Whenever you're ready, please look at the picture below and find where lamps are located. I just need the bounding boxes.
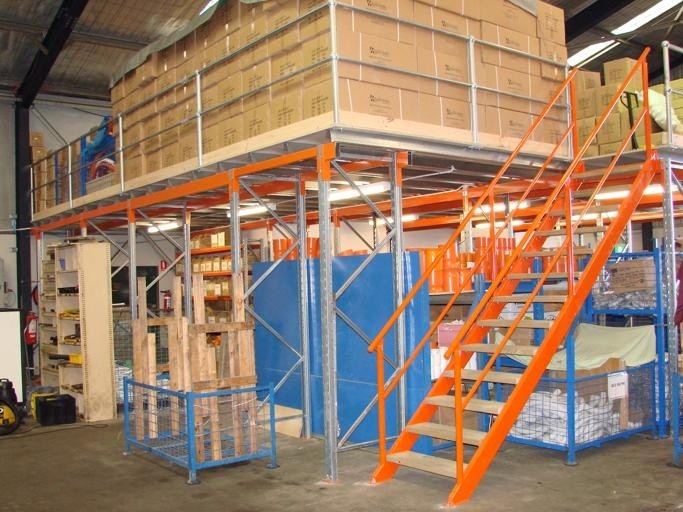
[470,218,527,231]
[326,180,392,202]
[592,182,680,202]
[570,208,621,223]
[473,198,531,214]
[148,218,184,233]
[225,200,277,219]
[368,213,421,227]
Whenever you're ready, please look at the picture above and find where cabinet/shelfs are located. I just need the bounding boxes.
[494,248,683,465]
[39,242,118,422]
[176,243,261,301]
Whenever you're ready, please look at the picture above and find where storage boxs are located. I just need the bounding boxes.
[550,354,683,423]
[30,0,683,211]
[31,390,77,426]
[174,227,257,324]
[609,256,656,295]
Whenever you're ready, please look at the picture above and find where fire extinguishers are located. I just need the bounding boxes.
[160,290,171,315]
[24,311,38,345]
